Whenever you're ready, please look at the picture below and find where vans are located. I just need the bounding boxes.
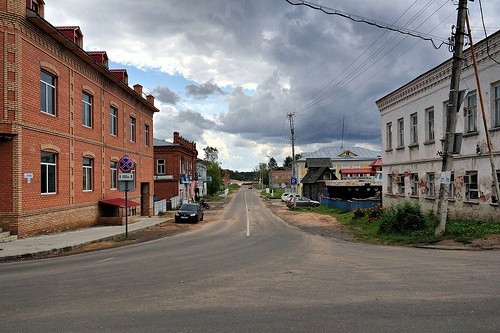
[281,193,300,201]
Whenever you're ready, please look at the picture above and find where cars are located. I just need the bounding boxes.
[174,204,204,224]
[248,186,252,189]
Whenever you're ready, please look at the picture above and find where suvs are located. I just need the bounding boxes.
[287,197,321,210]
